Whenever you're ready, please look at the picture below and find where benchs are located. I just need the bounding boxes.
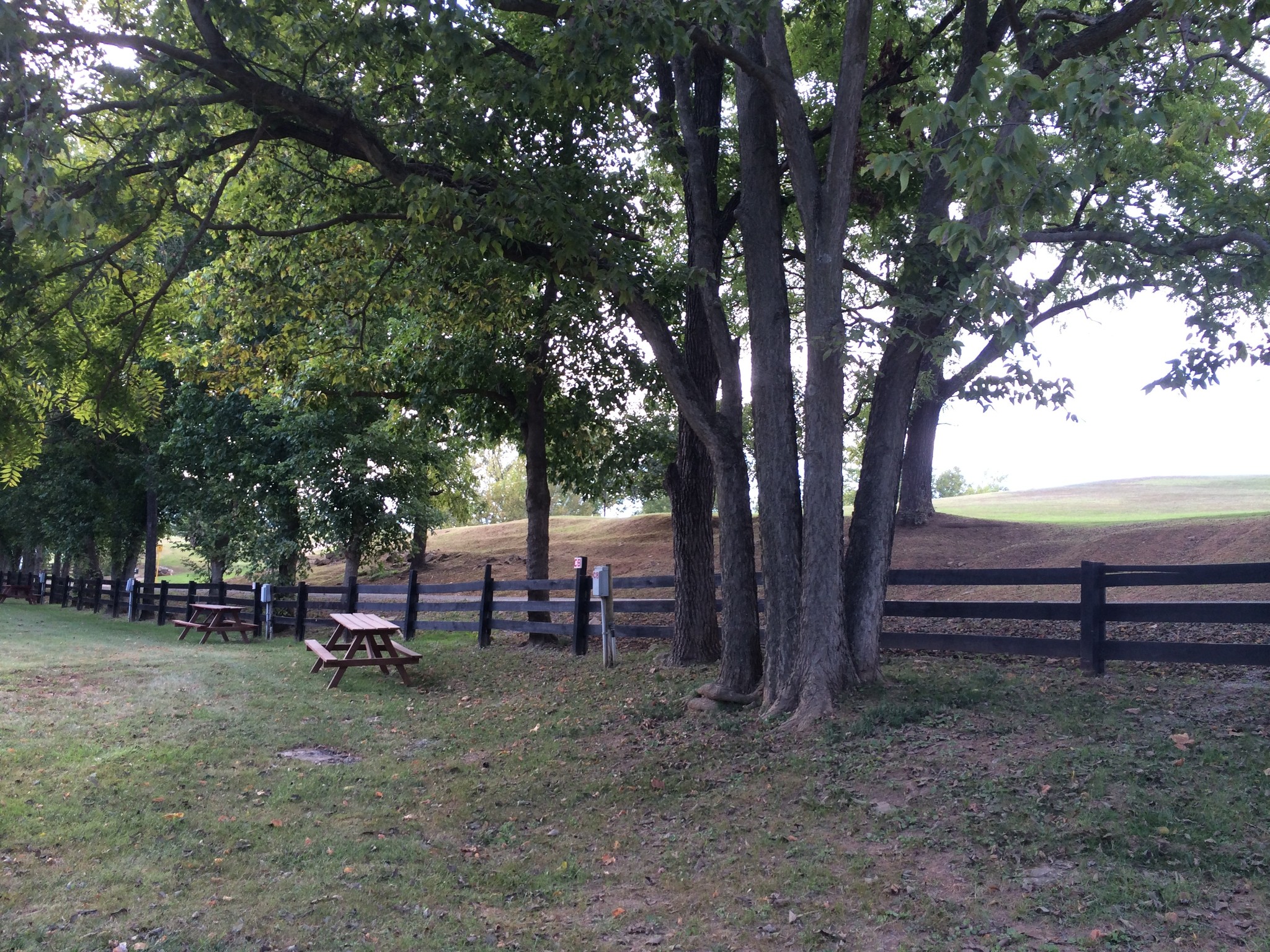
[0,592,8,598]
[171,619,208,632]
[388,640,423,661]
[302,639,341,667]
[31,592,42,598]
[222,619,260,630]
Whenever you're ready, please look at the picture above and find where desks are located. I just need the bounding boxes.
[0,584,38,605]
[310,610,412,691]
[178,603,250,646]
